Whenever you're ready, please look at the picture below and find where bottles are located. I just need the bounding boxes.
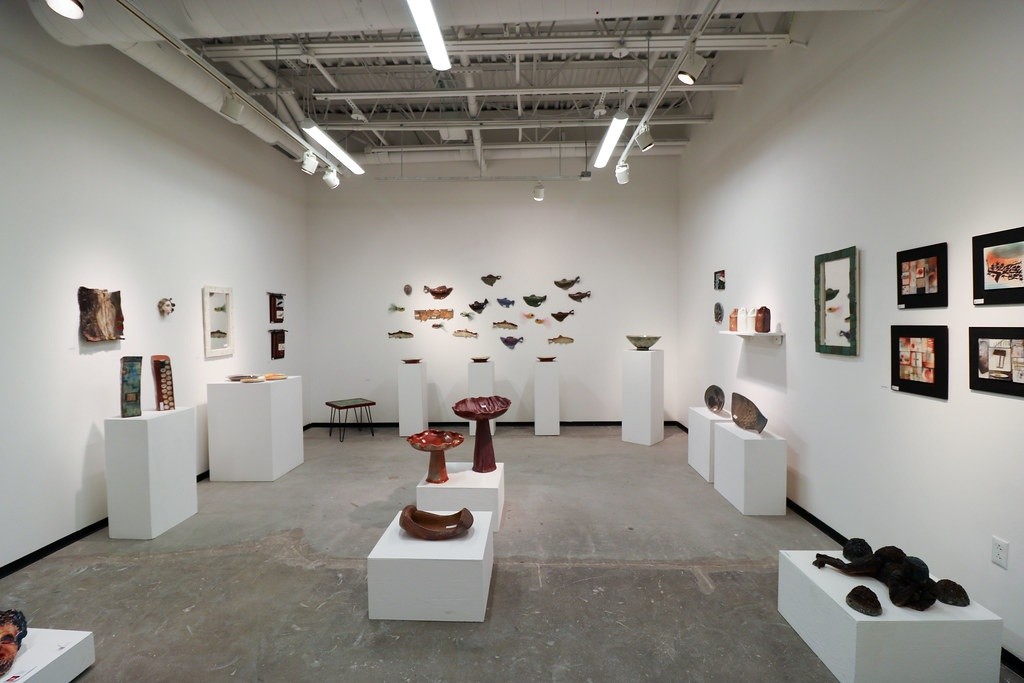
[729,306,770,333]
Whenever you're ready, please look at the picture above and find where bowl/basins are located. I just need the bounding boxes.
[626,335,662,351]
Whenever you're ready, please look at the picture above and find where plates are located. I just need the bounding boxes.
[704,385,725,413]
[226,373,288,382]
[537,356,556,362]
[402,358,421,364]
[471,357,489,363]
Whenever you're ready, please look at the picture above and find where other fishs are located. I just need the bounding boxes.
[547,335,574,345]
[569,291,592,302]
[500,336,524,348]
[524,312,545,324]
[481,274,501,287]
[496,297,515,308]
[388,304,405,313]
[493,320,519,330]
[553,276,579,290]
[453,330,478,338]
[522,294,547,307]
[468,299,489,314]
[387,330,413,339]
[424,285,452,296]
[432,324,443,330]
[551,310,574,323]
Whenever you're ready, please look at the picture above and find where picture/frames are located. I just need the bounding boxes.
[891,225,1023,400]
[814,246,859,356]
[203,286,236,358]
[713,270,725,290]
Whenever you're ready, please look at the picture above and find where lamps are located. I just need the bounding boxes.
[322,169,340,191]
[676,41,709,84]
[615,162,630,186]
[534,180,545,201]
[220,93,244,122]
[46,0,84,20]
[406,0,452,72]
[586,52,630,172]
[302,150,319,176]
[298,64,366,176]
[634,125,656,152]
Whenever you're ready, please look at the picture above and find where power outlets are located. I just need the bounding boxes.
[991,534,1010,570]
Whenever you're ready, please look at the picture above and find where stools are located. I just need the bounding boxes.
[325,398,376,442]
